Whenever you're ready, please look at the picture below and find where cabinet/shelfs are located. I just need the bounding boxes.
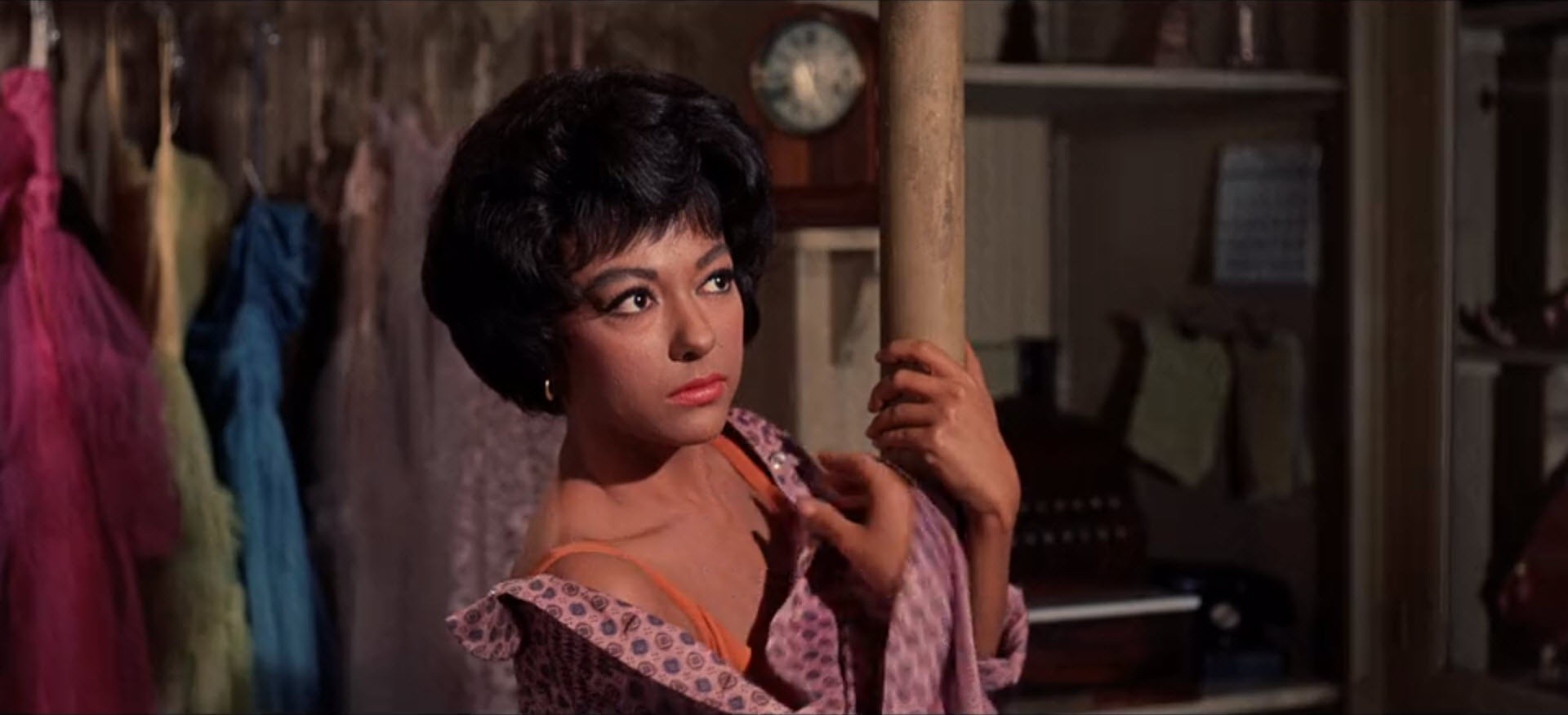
[969,52,1352,715]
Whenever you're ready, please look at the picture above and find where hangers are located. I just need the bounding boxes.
[19,0,624,230]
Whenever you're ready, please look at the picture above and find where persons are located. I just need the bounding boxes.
[421,59,1039,715]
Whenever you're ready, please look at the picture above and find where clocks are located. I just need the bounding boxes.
[731,16,889,198]
